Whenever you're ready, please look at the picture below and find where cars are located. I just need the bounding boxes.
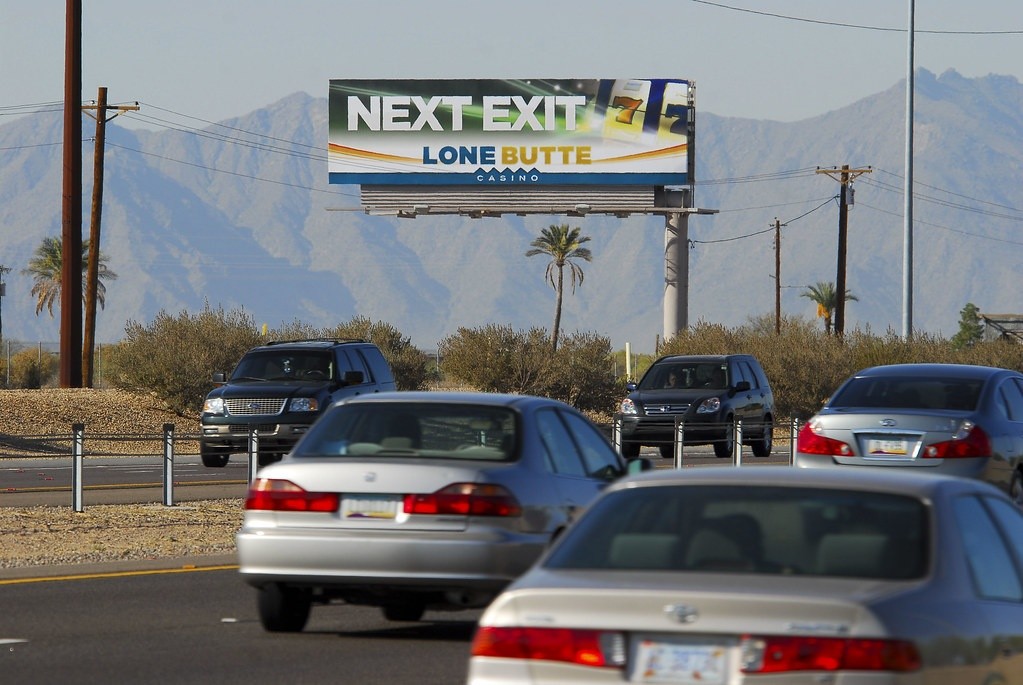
[470,469,1022,685]
[234,391,652,633]
[794,364,1023,489]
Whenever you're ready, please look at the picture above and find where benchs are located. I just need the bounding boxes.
[323,438,512,463]
[607,529,922,577]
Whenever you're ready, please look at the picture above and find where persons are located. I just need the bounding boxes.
[265,360,285,380]
[704,367,725,388]
[666,370,684,388]
[895,387,922,409]
[295,356,328,378]
[942,382,972,410]
[391,414,423,448]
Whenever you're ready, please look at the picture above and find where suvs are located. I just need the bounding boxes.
[614,354,775,461]
[199,338,400,469]
